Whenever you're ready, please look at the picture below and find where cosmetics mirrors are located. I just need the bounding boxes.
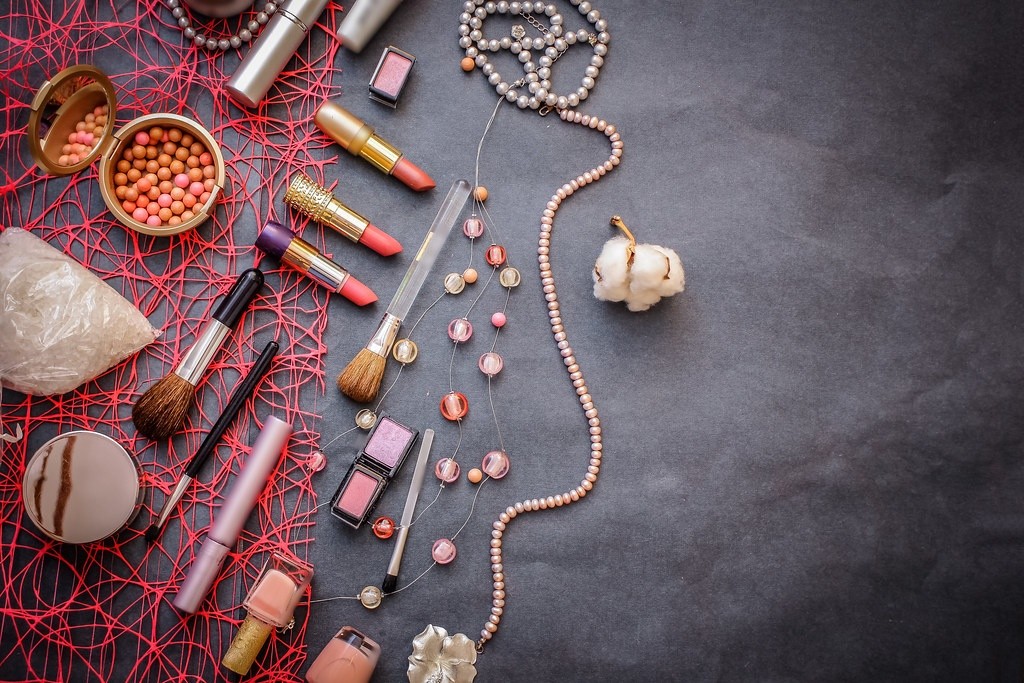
[28,65,116,177]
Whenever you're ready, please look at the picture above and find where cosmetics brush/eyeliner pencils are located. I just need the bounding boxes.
[336,179,472,403]
[381,428,435,594]
[132,267,280,545]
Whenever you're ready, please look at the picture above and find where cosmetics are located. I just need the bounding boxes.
[23,431,146,545]
[226,0,418,110]
[172,415,419,683]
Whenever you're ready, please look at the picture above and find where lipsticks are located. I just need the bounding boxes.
[254,102,437,307]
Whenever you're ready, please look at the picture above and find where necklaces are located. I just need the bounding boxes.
[306,0,624,683]
[166,0,284,49]
